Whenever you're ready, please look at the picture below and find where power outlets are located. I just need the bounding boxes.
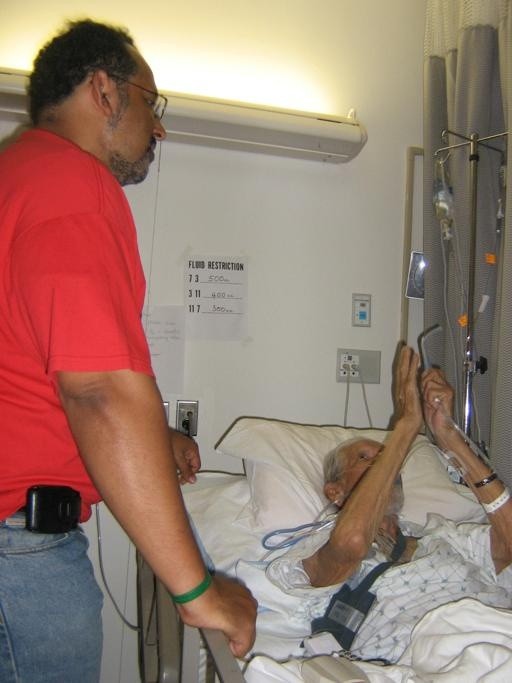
[336,348,382,386]
[176,400,200,438]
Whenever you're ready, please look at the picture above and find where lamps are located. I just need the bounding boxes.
[0,61,367,166]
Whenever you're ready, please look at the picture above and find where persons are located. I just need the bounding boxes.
[0,20,258,683]
[266,345,512,683]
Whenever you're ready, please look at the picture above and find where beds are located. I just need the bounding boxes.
[133,412,511,682]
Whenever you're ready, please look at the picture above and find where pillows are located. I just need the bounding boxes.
[214,416,491,537]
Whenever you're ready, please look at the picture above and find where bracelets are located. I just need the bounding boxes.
[172,570,213,604]
[475,472,497,487]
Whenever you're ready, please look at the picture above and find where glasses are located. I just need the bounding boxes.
[108,71,167,120]
